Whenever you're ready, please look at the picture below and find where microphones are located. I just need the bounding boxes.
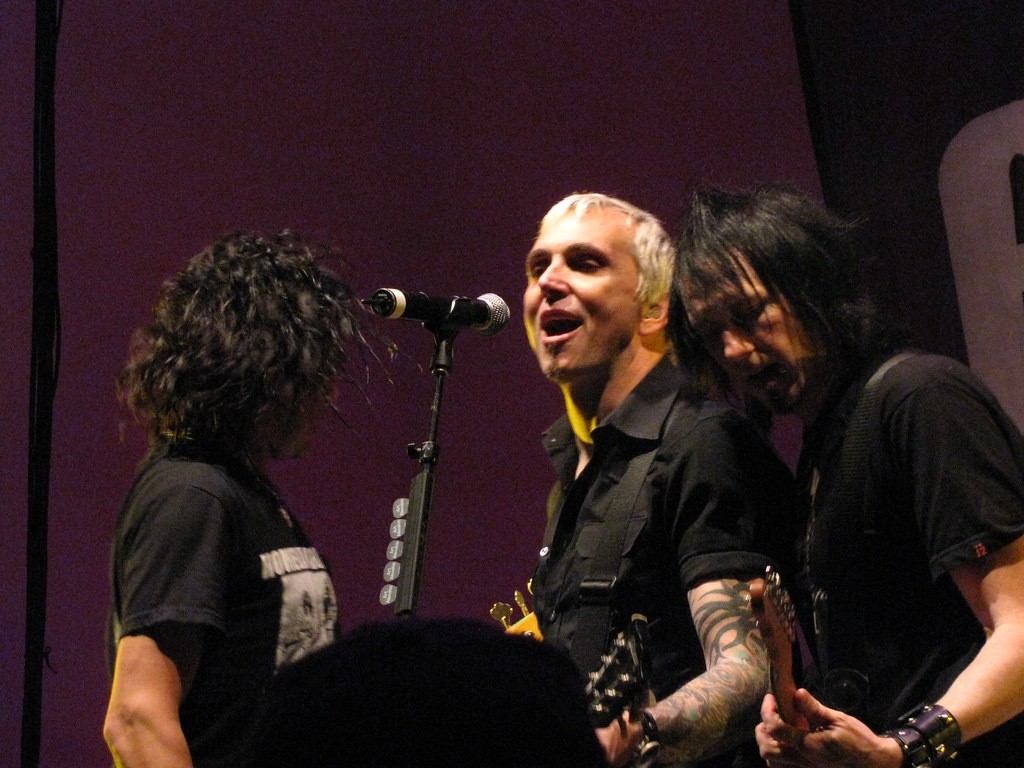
[361,289,511,336]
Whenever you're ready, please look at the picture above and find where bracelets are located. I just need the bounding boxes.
[883,703,961,768]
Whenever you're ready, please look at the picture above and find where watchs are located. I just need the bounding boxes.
[635,710,661,768]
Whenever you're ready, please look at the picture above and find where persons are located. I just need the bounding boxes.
[663,180,1024,768]
[521,192,794,768]
[101,228,370,768]
[250,616,605,768]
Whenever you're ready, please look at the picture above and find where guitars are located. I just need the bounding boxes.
[748,564,823,732]
[579,613,649,726]
